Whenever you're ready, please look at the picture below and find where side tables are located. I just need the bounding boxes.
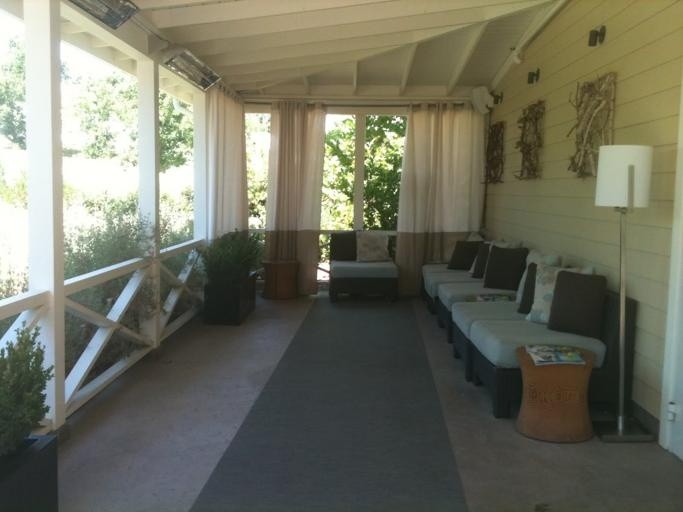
[514,345,596,443]
[260,260,300,299]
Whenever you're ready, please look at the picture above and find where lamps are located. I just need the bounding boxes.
[161,43,222,92]
[528,69,540,84]
[592,145,655,443]
[589,26,606,46]
[494,92,503,104]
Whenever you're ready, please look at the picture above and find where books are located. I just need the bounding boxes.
[525,342,587,369]
[475,291,510,303]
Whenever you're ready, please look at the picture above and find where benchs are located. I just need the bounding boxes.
[330,231,397,302]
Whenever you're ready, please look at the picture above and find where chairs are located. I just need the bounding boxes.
[470,272,637,418]
[451,252,595,382]
[424,232,531,347]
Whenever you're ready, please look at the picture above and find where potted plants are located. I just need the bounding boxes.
[203,229,267,324]
[0,320,57,512]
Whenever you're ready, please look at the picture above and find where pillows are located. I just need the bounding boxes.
[356,232,390,261]
[516,262,538,315]
[526,262,593,325]
[525,249,562,270]
[545,271,610,341]
